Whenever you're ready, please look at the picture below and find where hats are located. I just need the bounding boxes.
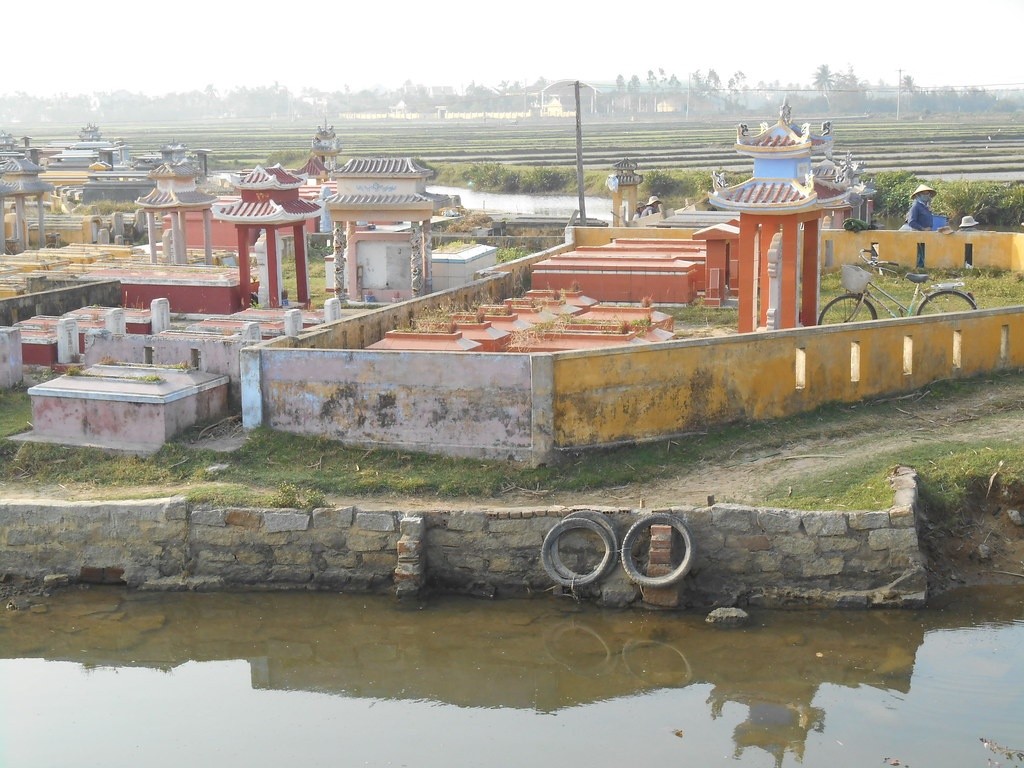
[636,202,645,212]
[645,196,663,207]
[959,215,979,227]
[905,212,909,221]
[911,184,936,199]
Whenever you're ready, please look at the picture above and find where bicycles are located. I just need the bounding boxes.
[816,249,978,327]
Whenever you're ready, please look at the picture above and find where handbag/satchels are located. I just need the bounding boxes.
[842,218,871,233]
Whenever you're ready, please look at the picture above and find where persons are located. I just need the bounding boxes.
[706,200,718,211]
[632,194,663,221]
[899,183,937,230]
[958,215,979,231]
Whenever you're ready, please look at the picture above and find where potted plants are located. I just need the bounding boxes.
[392,291,402,303]
[281,289,288,307]
[364,290,374,302]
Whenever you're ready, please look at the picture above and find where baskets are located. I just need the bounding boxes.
[841,264,872,292]
